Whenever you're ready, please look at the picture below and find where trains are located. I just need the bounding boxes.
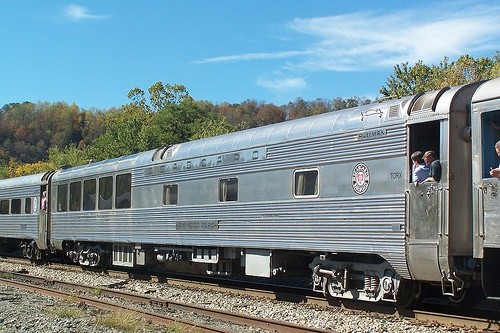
[0,76,500,308]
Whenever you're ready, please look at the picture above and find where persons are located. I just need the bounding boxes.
[489,140,500,178]
[41,191,46,210]
[410,152,431,187]
[423,151,442,184]
